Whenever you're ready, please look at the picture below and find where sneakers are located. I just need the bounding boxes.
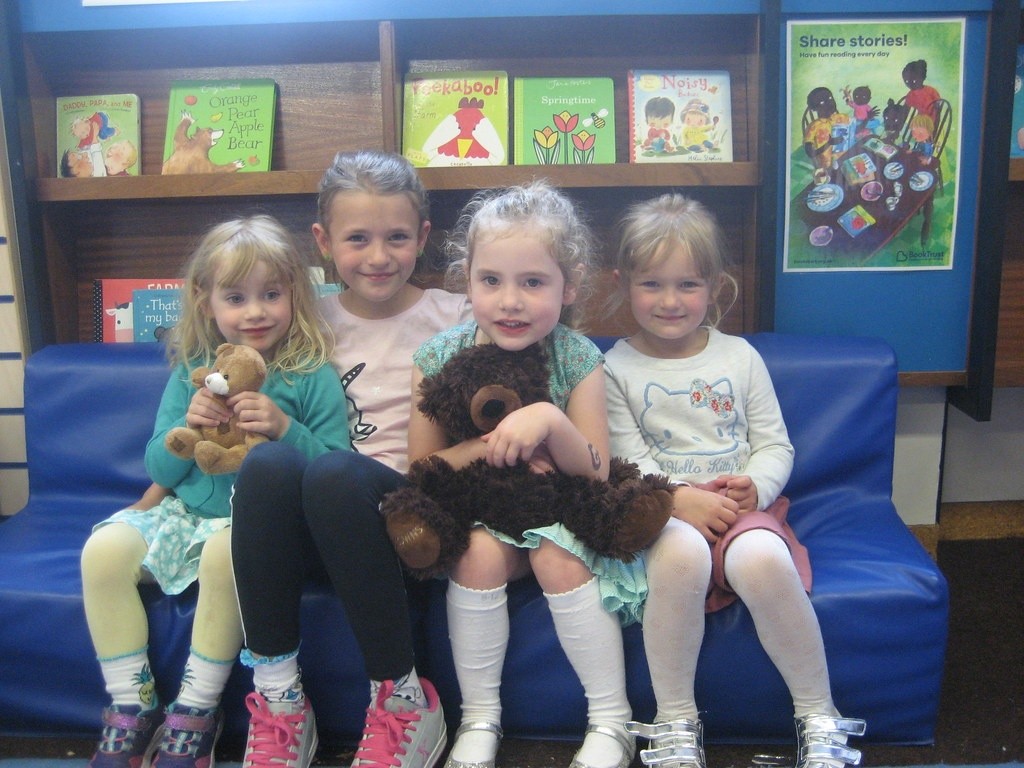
[350,675,448,768]
[624,719,707,768]
[242,690,318,768]
[87,702,167,768]
[794,704,867,768]
[151,702,226,768]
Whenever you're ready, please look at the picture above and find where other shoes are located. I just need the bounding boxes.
[442,721,504,768]
[569,724,637,768]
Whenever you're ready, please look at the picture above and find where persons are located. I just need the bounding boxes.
[598,193,868,768]
[78,214,348,768]
[231,147,475,768]
[406,177,636,768]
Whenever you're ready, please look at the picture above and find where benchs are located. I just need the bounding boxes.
[0,330,954,752]
